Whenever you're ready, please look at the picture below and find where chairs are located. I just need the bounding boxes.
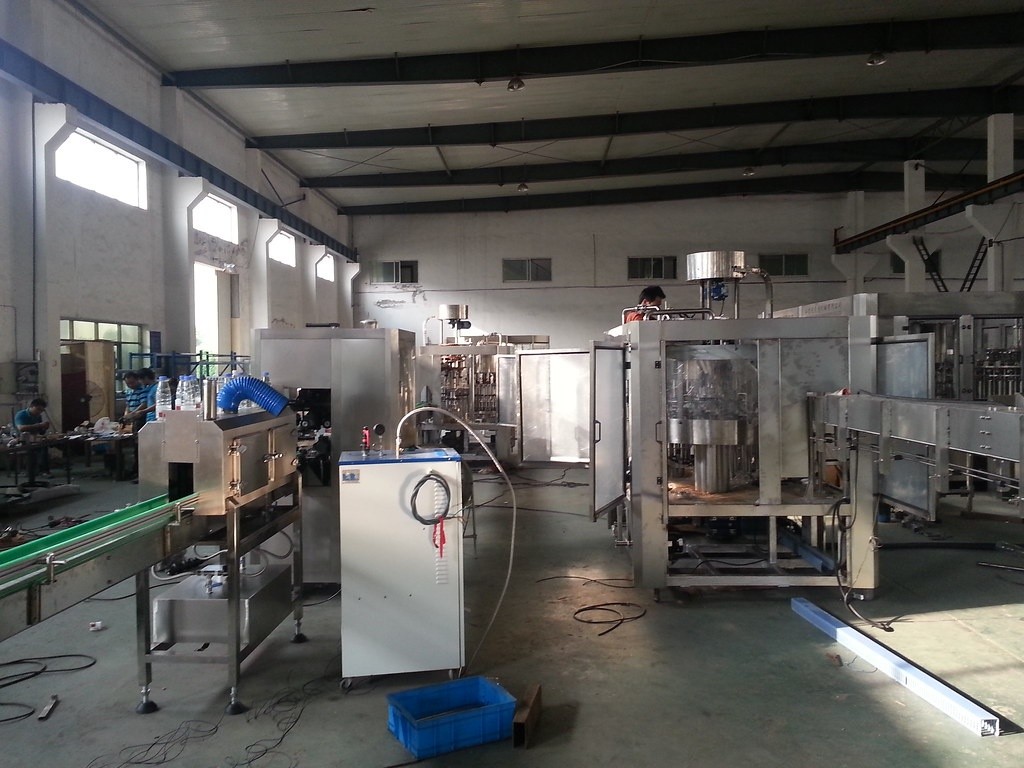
[5,406,51,484]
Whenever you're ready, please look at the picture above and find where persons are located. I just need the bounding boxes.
[14,398,54,479]
[625,285,666,323]
[118,368,159,484]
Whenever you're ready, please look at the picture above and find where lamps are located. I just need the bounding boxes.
[518,184,529,192]
[507,79,525,92]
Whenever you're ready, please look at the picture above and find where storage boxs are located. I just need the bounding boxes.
[386,675,517,760]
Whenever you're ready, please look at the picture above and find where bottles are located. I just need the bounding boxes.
[176,375,201,410]
[155,376,172,420]
[224,371,271,409]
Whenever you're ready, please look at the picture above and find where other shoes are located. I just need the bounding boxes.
[130,472,138,479]
[42,472,54,478]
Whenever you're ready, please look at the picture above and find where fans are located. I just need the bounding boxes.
[64,380,104,421]
